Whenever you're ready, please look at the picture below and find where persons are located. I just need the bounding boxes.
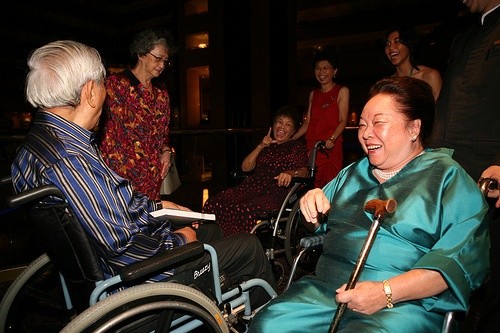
[199,108,310,235]
[99,29,174,200]
[0,0,500,333]
[291,56,349,188]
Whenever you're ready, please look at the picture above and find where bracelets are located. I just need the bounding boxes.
[292,168,298,177]
[329,136,335,142]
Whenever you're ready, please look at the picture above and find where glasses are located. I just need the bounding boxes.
[314,66,333,72]
[145,48,170,66]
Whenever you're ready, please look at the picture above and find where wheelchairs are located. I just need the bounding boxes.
[227,140,333,274]
[285,178,499,333]
[0,175,281,333]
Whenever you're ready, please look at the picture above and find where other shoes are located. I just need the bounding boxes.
[273,261,284,286]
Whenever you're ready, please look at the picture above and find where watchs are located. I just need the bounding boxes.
[162,150,173,160]
[382,278,394,308]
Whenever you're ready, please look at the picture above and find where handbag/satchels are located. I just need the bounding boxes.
[160,150,182,195]
